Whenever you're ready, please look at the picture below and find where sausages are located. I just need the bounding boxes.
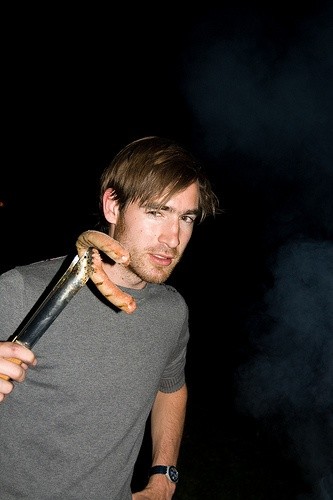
[75,229,139,315]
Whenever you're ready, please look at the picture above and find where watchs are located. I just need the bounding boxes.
[149,464,182,484]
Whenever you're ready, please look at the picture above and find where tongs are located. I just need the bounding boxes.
[0,247,95,380]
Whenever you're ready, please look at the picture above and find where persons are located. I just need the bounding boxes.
[1,134,225,500]
